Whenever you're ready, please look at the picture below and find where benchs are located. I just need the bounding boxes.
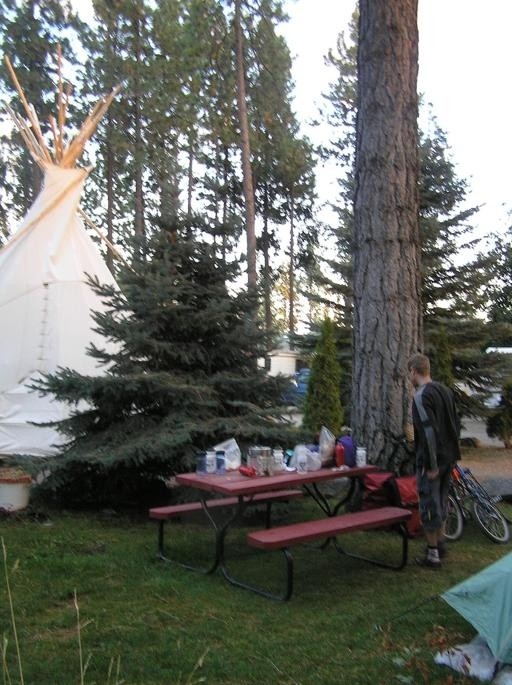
[242,507,418,603]
[148,489,311,575]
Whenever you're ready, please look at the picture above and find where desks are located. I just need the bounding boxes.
[173,460,380,576]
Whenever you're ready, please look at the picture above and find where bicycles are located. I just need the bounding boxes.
[399,437,511,545]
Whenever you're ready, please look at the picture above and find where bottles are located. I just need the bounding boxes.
[196,425,367,475]
[452,468,460,480]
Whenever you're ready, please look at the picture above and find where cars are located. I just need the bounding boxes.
[277,368,311,407]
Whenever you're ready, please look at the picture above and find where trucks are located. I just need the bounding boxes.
[454,346,512,449]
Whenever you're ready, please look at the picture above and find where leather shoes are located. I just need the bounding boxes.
[419,541,447,558]
[413,554,442,570]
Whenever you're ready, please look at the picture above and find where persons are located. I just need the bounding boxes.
[406,355,462,568]
